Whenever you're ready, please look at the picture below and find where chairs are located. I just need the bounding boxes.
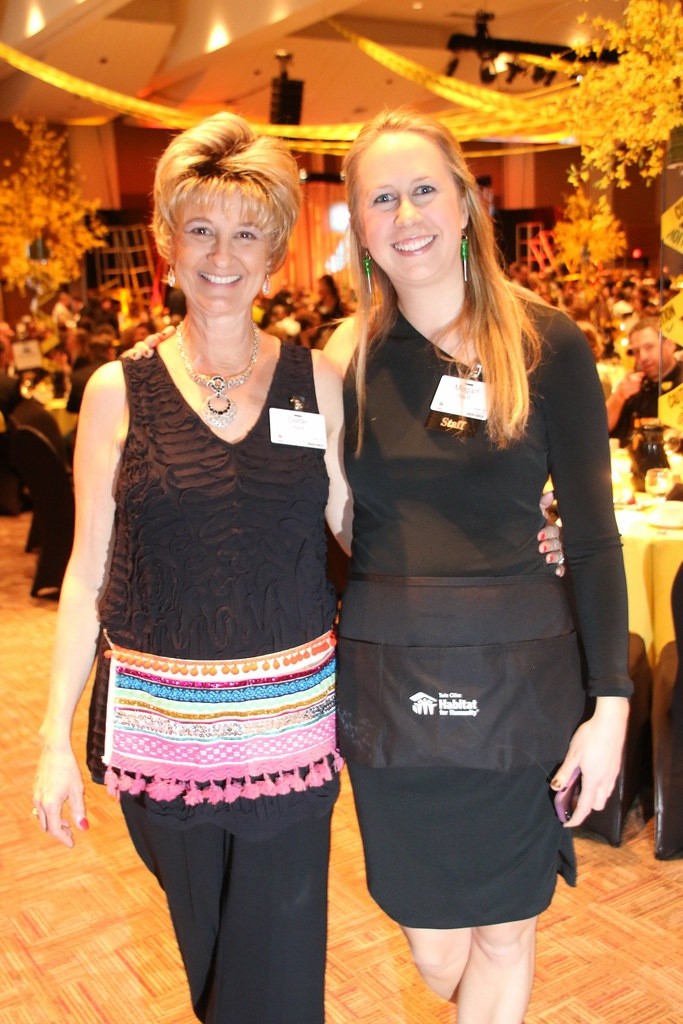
[4,423,74,598]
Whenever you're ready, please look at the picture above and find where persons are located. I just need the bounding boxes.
[0,271,682,599]
[321,113,636,1024]
[31,111,573,1022]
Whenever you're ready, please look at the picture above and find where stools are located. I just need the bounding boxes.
[646,641,683,859]
[576,632,653,847]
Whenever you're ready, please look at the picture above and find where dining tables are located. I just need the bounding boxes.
[607,490,682,669]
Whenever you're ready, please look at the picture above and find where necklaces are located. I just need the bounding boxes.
[173,320,259,424]
